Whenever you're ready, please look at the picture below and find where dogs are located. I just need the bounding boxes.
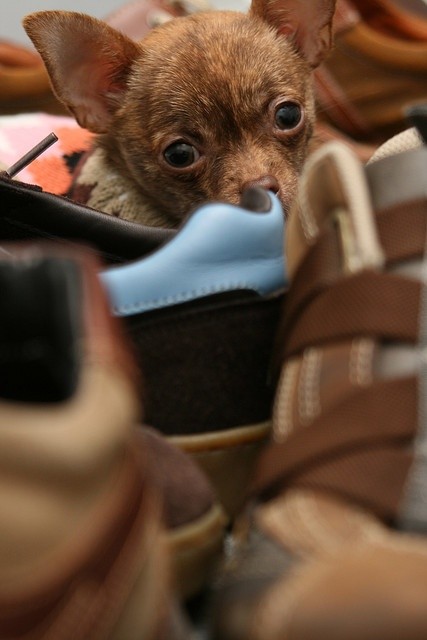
[23,2,339,227]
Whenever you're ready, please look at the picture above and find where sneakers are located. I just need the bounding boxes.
[0,131,287,526]
[0,248,229,639]
[212,126,426,638]
